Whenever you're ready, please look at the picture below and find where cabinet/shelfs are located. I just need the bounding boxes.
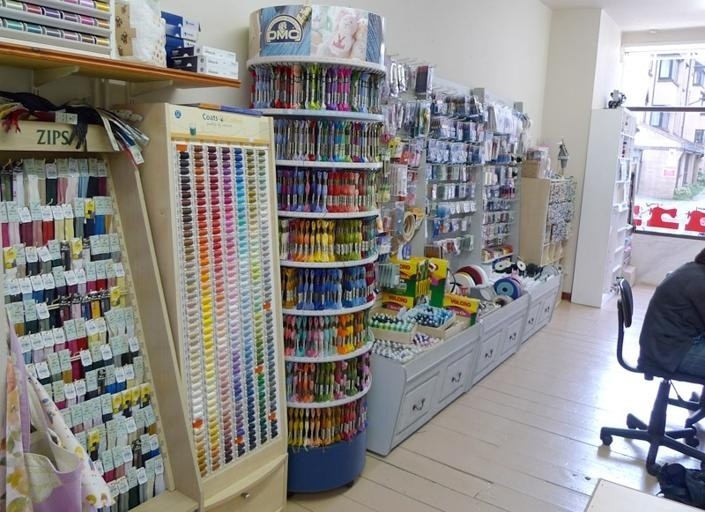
[359,273,563,459]
[519,106,639,309]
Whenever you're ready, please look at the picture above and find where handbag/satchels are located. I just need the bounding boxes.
[656,464,705,509]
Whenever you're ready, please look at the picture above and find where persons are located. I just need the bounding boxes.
[636,248,705,377]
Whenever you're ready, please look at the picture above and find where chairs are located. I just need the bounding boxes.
[599,278,705,478]
